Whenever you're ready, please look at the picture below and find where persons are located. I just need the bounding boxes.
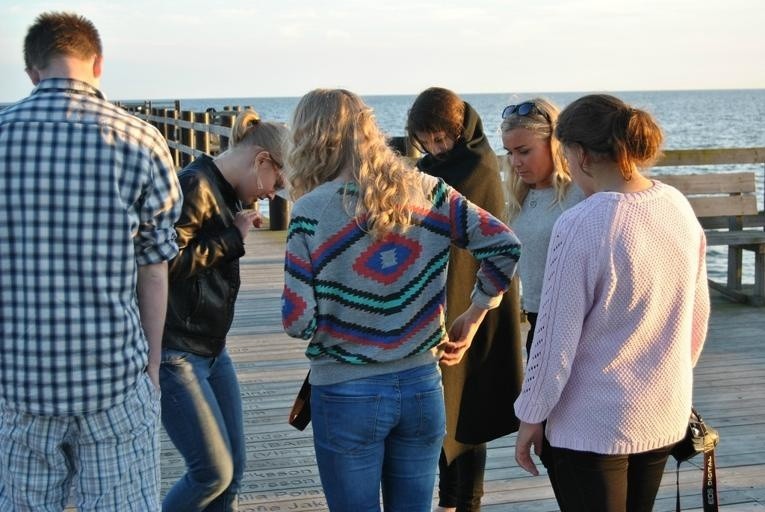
[160,107,293,509]
[280,90,524,512]
[408,89,525,512]
[513,94,710,512]
[0,10,180,510]
[499,98,588,365]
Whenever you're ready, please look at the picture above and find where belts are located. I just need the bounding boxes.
[289,368,311,430]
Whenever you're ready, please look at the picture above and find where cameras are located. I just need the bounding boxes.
[674,418,719,464]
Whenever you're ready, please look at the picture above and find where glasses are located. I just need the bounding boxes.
[502,103,543,119]
